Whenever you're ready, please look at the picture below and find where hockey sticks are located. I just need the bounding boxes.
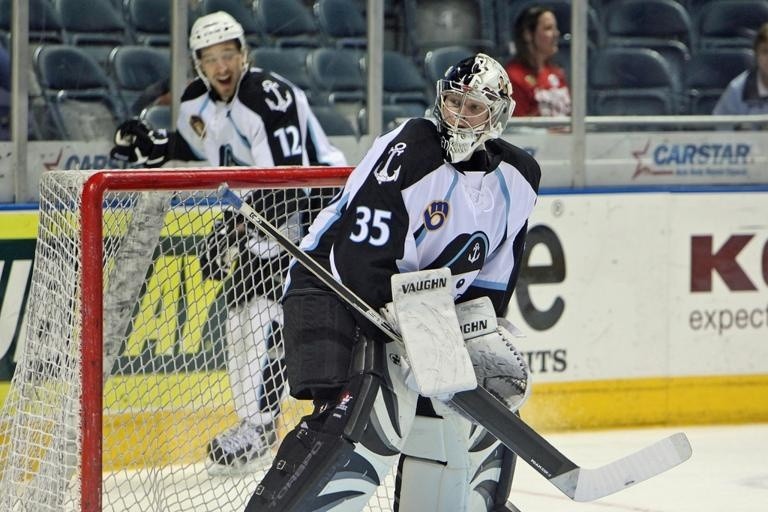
[219,183,693,502]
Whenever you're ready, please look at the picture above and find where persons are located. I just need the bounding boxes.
[113,11,348,465]
[711,24,768,130]
[243,53,542,512]
[504,5,571,119]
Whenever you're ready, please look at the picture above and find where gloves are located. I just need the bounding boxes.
[201,210,255,282]
[110,118,169,168]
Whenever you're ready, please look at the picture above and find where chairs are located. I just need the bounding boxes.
[585,0,767,132]
[383,1,573,137]
[1,0,369,137]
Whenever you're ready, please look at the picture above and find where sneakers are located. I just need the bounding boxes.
[207,417,277,467]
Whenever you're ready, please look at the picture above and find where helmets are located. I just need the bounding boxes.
[188,11,247,93]
[432,53,516,164]
